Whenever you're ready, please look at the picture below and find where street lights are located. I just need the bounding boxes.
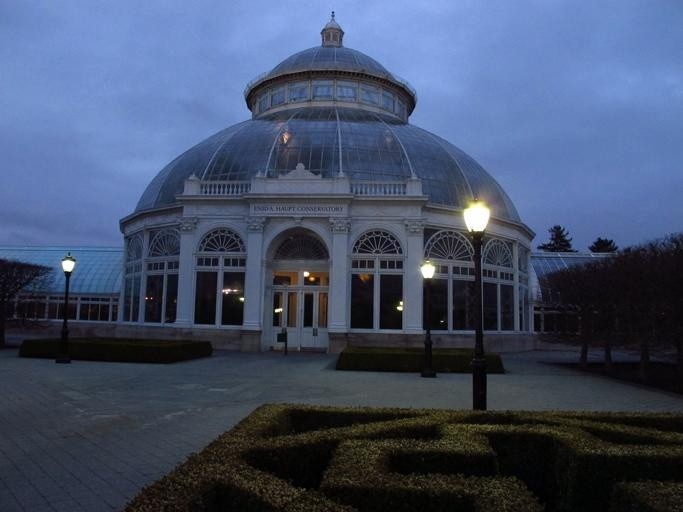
[417,259,437,378]
[461,192,493,410]
[55,251,78,364]
